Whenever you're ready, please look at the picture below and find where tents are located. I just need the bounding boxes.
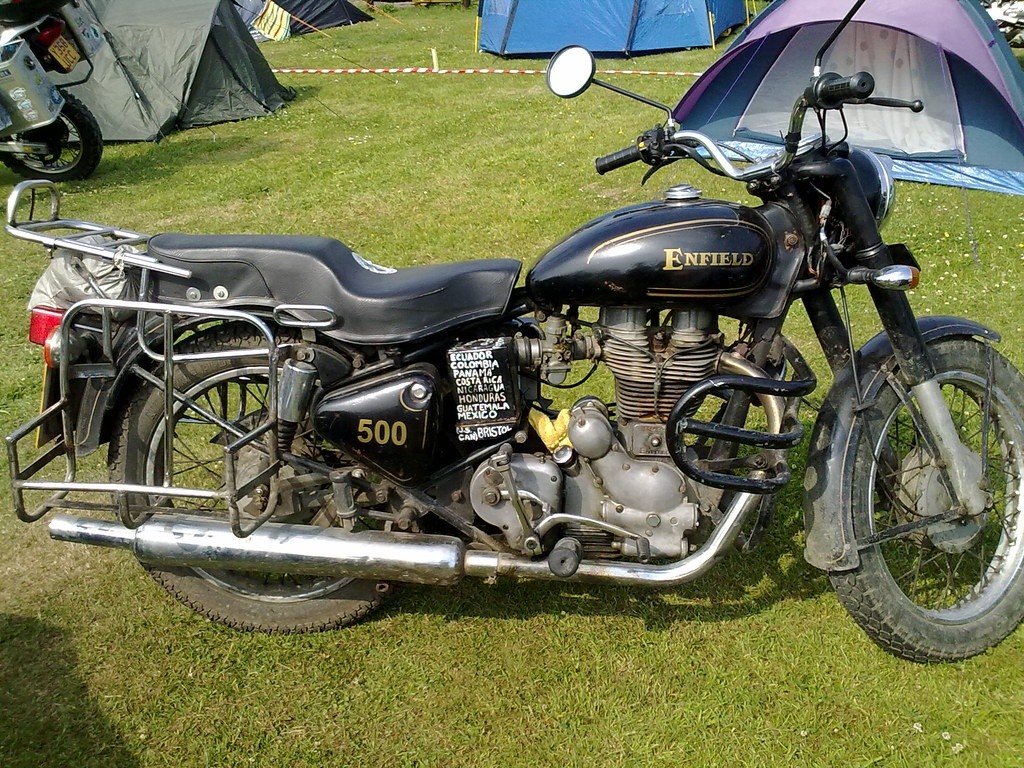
[0,0,297,144]
[232,0,376,43]
[659,0,1024,195]
[478,0,755,55]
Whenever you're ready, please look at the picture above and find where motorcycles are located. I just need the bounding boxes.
[0,0,1024,666]
[0,0,103,183]
